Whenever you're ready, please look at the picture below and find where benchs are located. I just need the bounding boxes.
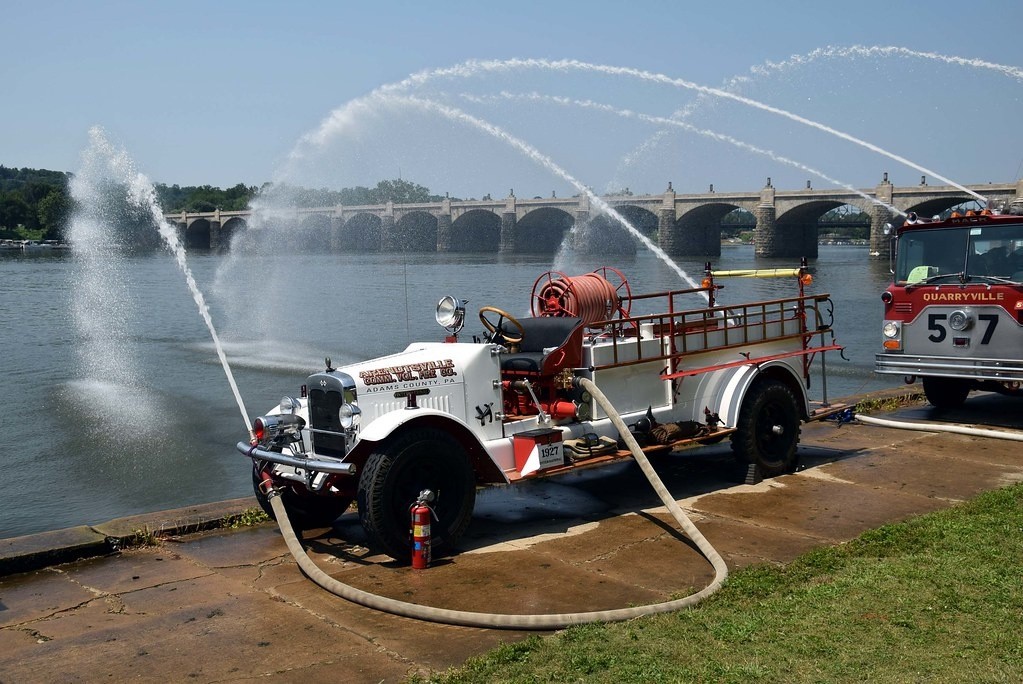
[486,316,585,402]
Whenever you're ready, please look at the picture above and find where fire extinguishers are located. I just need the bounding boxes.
[406,490,440,568]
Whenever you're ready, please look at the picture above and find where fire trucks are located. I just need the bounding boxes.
[237,262,851,557]
[875,195,1023,415]
